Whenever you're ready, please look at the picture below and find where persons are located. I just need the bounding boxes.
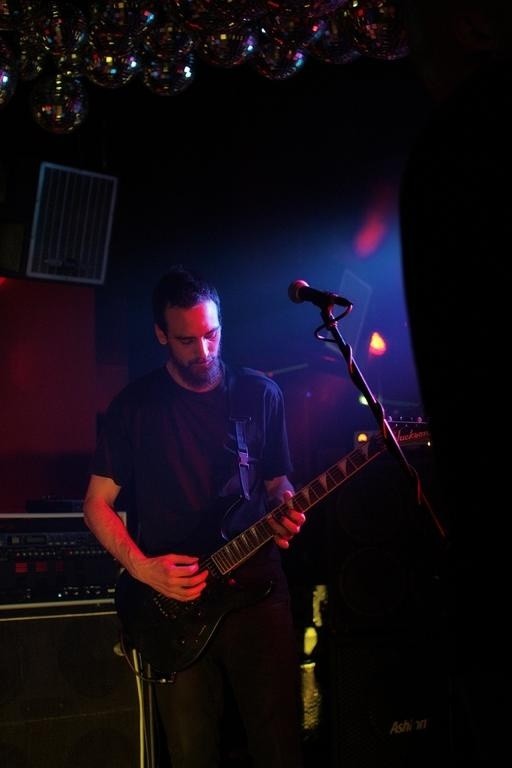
[82,270,306,768]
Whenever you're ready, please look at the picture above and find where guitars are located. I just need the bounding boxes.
[115,415,431,676]
